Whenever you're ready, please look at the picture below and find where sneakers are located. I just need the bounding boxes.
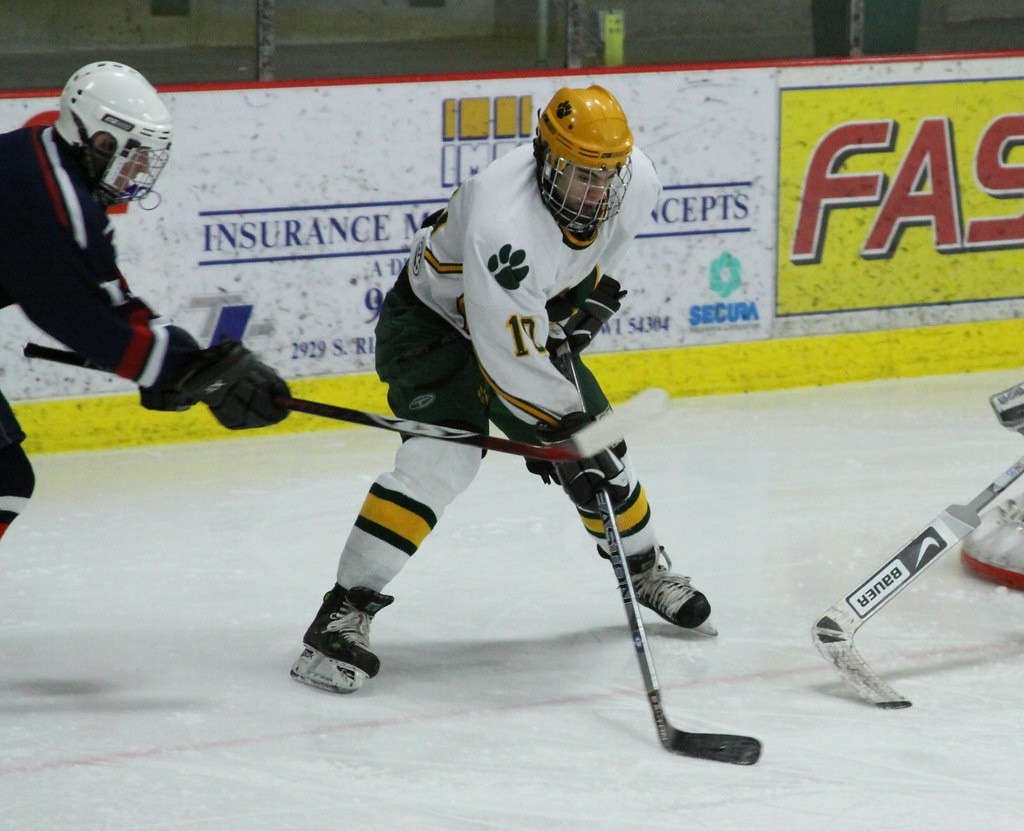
[632,545,718,636]
[290,580,395,695]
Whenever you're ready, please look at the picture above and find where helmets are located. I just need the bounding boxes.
[532,85,632,173]
[54,59,173,162]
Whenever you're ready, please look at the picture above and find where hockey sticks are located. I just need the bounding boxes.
[810,457,1024,708]
[20,341,670,463]
[551,341,763,767]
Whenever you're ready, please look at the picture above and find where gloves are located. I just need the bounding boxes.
[139,326,200,412]
[550,271,628,363]
[536,411,630,514]
[194,340,294,431]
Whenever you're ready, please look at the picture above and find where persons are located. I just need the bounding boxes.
[0,63,293,538]
[288,83,720,694]
